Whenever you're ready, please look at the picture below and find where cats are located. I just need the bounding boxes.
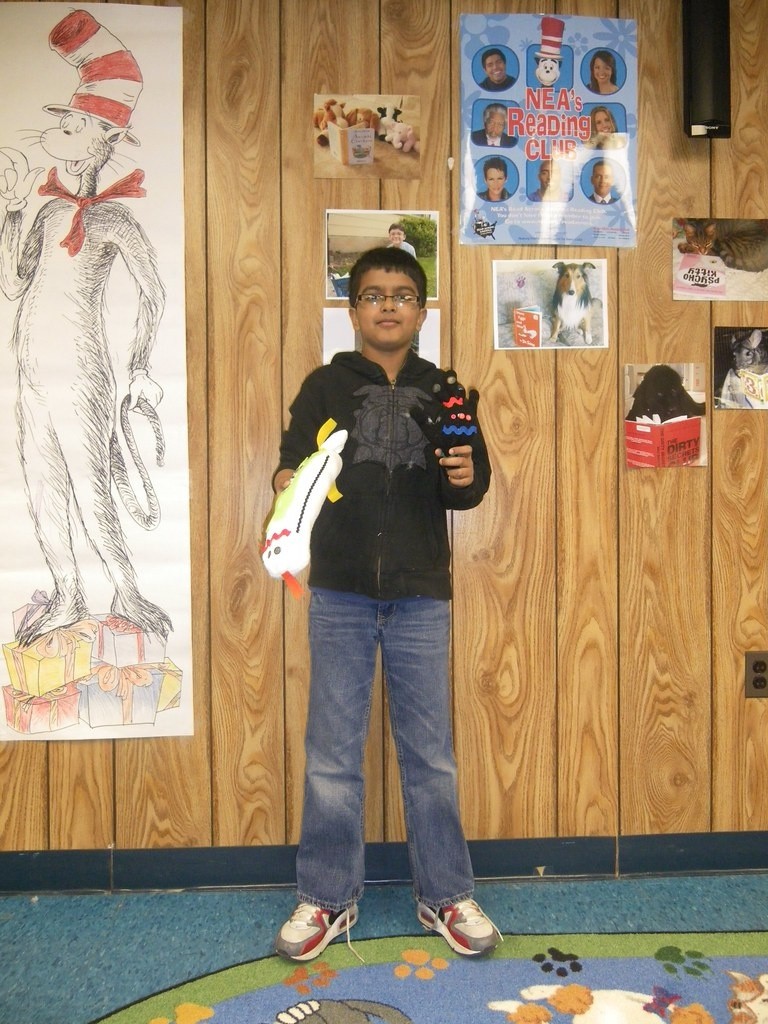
[678,218,768,274]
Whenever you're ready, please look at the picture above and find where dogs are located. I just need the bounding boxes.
[625,364,706,423]
[548,261,597,345]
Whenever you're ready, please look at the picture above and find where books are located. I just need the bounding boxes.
[740,369,768,404]
[514,305,542,347]
[326,121,374,164]
[331,272,351,298]
[625,414,701,468]
[673,253,727,297]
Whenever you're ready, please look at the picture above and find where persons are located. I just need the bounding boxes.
[271,246,503,962]
[589,160,624,204]
[478,48,517,92]
[472,103,518,148]
[586,50,619,94]
[583,106,626,150]
[529,159,570,202]
[478,157,512,201]
[386,223,416,259]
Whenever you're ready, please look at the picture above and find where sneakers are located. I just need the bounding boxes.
[274,903,368,963]
[417,898,504,959]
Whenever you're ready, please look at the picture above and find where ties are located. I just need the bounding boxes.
[602,200,606,204]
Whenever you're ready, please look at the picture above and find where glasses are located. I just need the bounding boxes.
[353,294,423,309]
[486,120,505,128]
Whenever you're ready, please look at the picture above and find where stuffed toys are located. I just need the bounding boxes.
[262,419,348,601]
[411,370,480,470]
[313,98,420,153]
